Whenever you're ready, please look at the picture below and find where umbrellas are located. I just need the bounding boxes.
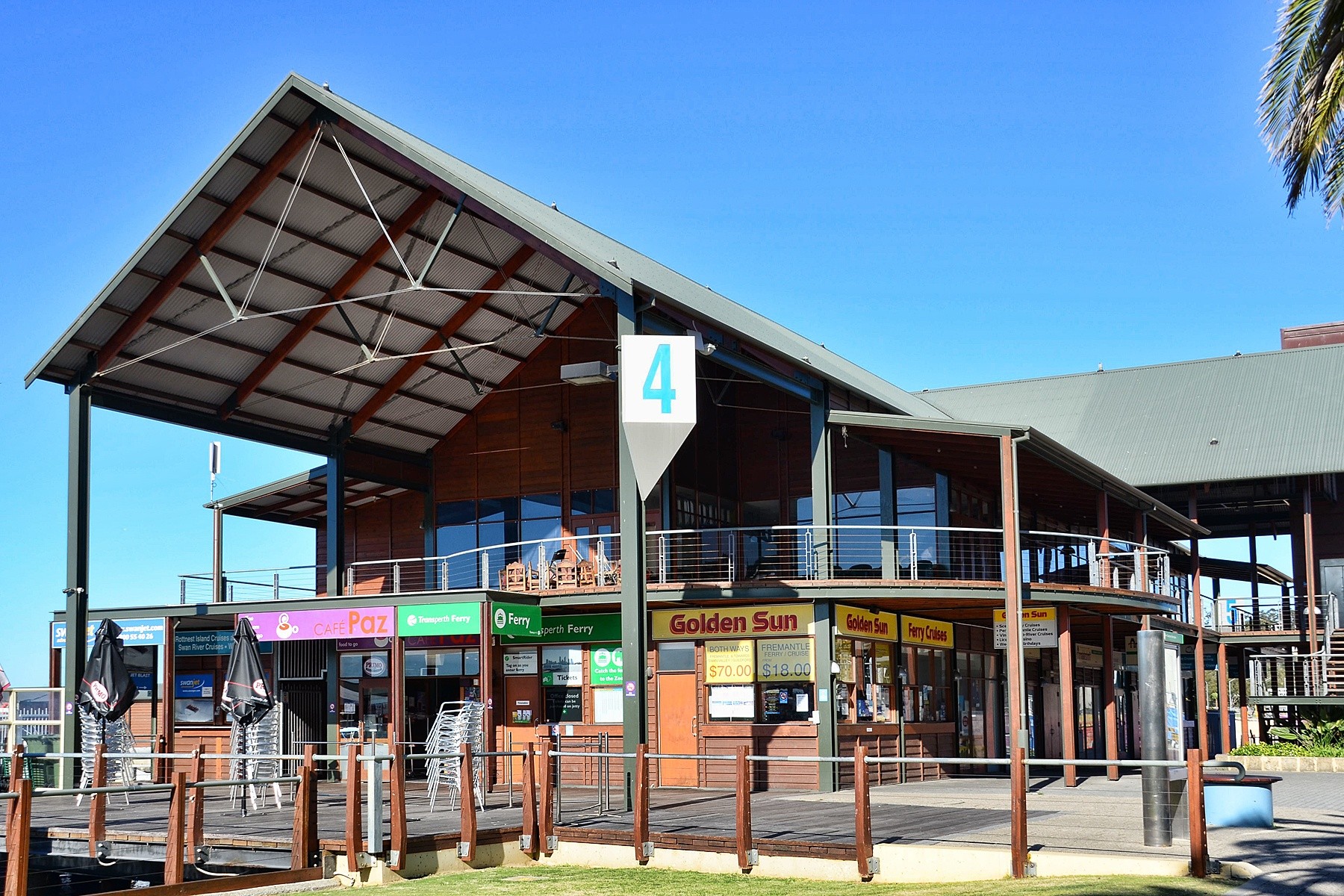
[221,616,277,816]
[69,617,142,750]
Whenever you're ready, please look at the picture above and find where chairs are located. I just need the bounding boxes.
[74,702,140,805]
[227,702,285,812]
[424,699,487,814]
[497,549,622,592]
